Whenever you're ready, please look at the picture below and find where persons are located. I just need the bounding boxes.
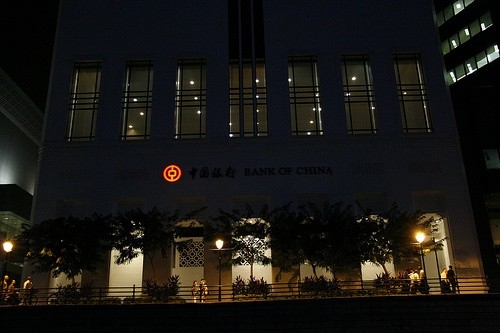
[191,279,208,303]
[441,264,456,294]
[0,275,33,306]
[398,265,425,294]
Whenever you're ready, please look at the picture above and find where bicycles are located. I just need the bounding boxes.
[46,284,79,304]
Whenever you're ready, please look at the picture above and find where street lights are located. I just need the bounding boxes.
[0,242,13,297]
[414,233,428,296]
[216,240,223,302]
